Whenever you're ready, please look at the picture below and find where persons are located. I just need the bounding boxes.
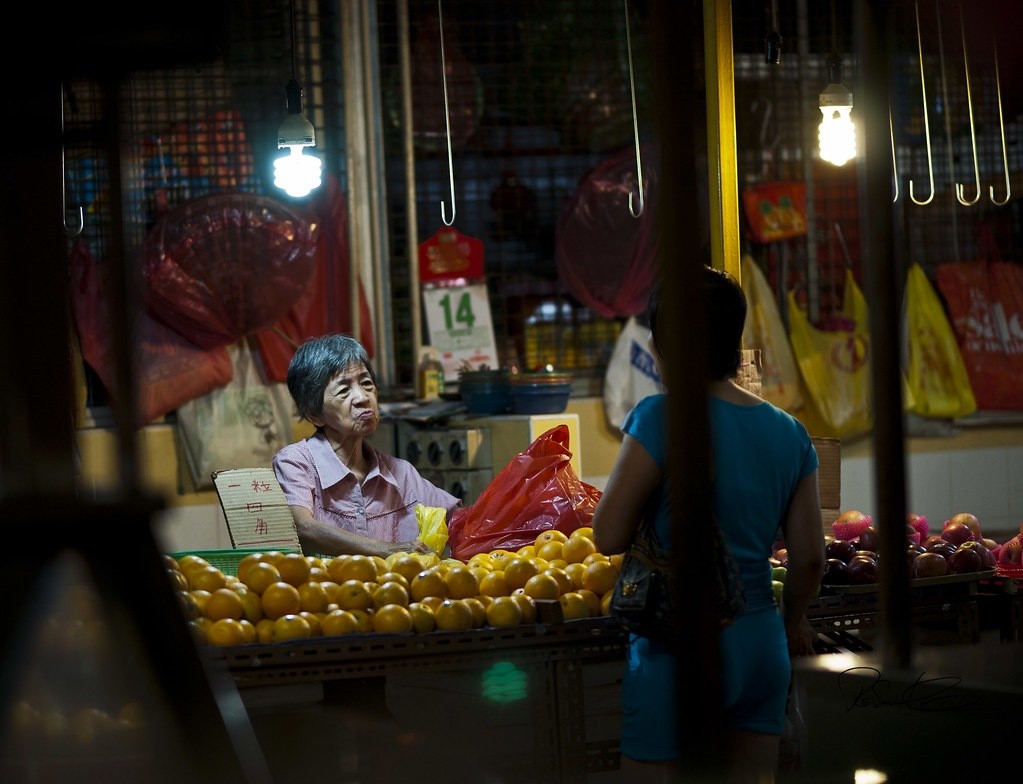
[594,264,829,784]
[271,333,465,558]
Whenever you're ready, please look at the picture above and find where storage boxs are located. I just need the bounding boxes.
[810,435,843,536]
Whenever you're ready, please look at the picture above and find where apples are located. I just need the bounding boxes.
[771,508,1023,600]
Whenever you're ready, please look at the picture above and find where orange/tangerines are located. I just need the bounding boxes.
[162,527,622,646]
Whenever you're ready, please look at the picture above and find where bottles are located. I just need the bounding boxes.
[421,352,444,398]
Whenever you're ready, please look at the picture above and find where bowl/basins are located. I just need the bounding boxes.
[510,373,573,414]
